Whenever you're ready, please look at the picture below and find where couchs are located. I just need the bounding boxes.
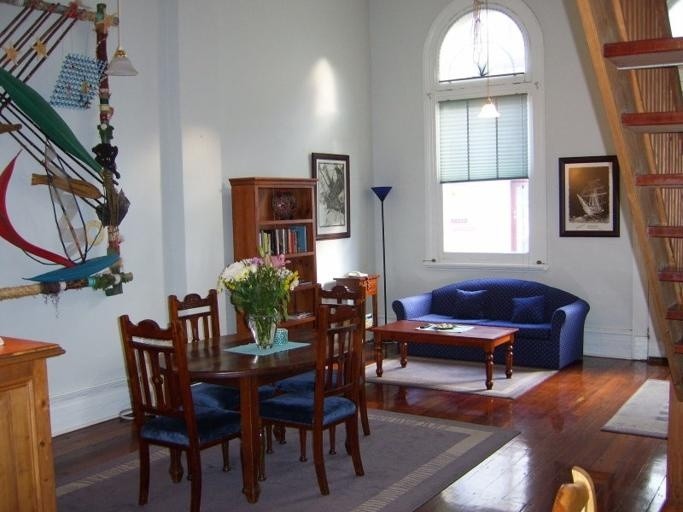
[391,278,589,364]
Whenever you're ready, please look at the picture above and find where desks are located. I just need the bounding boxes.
[334,275,380,336]
[0,334,67,512]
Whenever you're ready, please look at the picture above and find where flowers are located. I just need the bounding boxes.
[215,253,301,343]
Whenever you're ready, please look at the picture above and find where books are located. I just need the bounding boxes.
[285,311,311,319]
[257,225,307,255]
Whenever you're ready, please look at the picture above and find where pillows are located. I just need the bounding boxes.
[509,293,545,323]
[452,285,489,319]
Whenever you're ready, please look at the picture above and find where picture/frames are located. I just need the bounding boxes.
[311,152,351,243]
[558,155,621,237]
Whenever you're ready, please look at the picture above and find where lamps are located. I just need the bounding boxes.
[371,186,393,324]
[476,1,501,119]
[102,1,139,77]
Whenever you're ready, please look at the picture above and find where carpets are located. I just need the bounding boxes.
[601,376,669,440]
[364,354,561,400]
[45,408,520,512]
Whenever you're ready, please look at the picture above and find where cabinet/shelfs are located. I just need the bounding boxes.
[229,177,319,330]
